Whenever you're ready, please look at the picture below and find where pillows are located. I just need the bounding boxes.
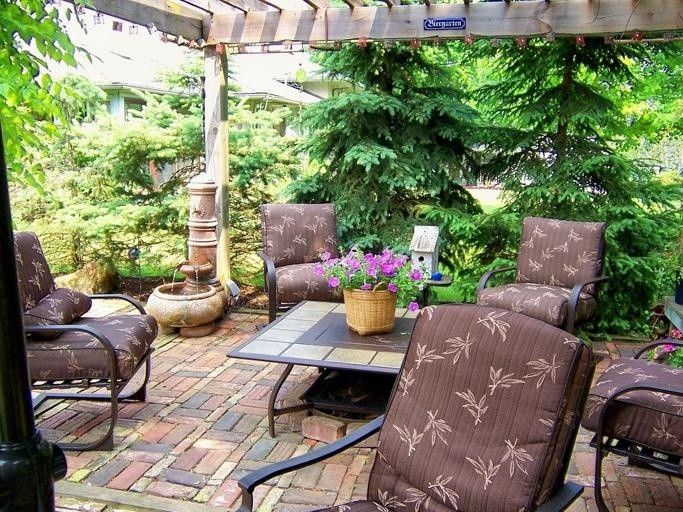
[24,287,92,340]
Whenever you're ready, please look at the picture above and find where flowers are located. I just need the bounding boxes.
[310,241,432,312]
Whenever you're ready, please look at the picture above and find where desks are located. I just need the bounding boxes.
[225,300,421,438]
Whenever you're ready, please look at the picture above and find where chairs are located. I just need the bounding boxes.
[256,204,344,332]
[475,217,614,335]
[13,231,158,451]
[231,303,596,512]
[581,339,683,512]
